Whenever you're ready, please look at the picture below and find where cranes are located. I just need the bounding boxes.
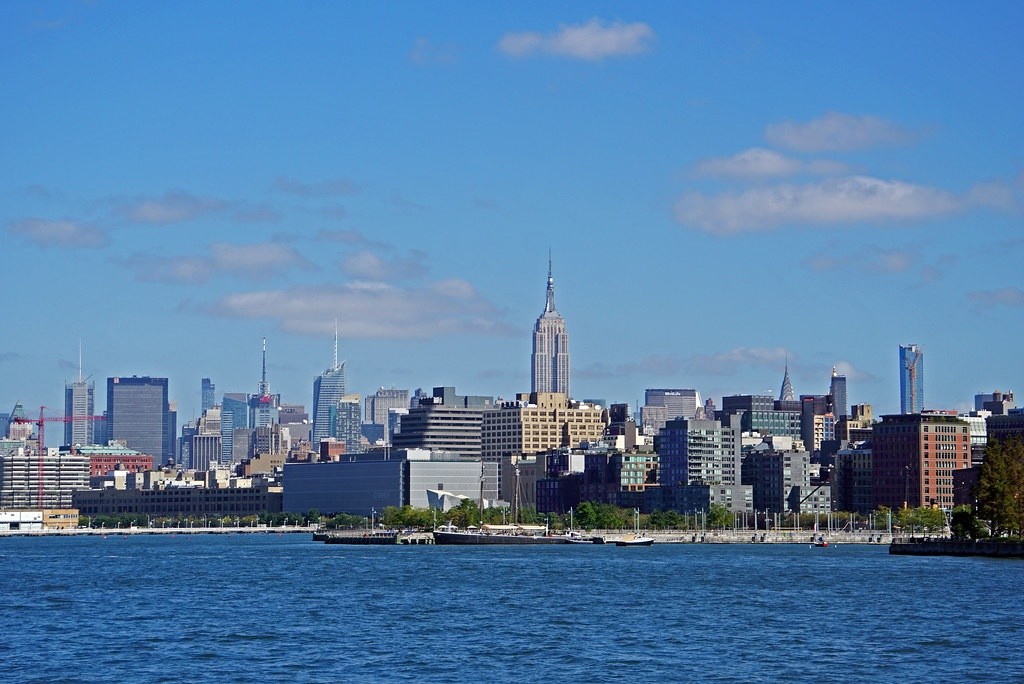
[13,405,110,509]
[905,342,926,414]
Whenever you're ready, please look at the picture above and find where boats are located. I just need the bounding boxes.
[565,536,594,544]
[815,540,829,547]
[592,531,655,546]
[433,450,568,545]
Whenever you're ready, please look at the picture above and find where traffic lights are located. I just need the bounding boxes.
[903,501,907,510]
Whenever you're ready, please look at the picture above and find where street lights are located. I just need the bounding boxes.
[118,522,121,531]
[209,521,211,528]
[190,520,193,529]
[130,522,133,531]
[295,520,299,528]
[269,521,273,526]
[251,521,254,528]
[222,521,224,529]
[162,521,166,530]
[101,521,105,531]
[238,521,240,529]
[177,522,181,530]
[308,520,310,528]
